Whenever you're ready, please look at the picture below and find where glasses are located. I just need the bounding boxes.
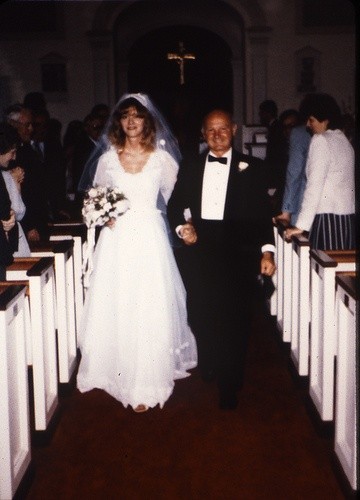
[11,119,33,129]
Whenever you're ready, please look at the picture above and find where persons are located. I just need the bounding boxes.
[257,99,290,213]
[0,167,32,259]
[272,85,321,229]
[23,88,109,222]
[282,93,359,251]
[281,107,360,174]
[73,92,199,413]
[0,119,22,281]
[165,103,277,410]
[0,103,52,250]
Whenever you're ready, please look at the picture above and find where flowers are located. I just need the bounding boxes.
[82,182,131,229]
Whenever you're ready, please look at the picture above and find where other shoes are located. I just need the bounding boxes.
[219,383,240,409]
[199,342,217,383]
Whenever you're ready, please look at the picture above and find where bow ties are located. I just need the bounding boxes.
[207,155,227,164]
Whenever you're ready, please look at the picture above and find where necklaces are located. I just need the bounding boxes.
[122,147,148,175]
